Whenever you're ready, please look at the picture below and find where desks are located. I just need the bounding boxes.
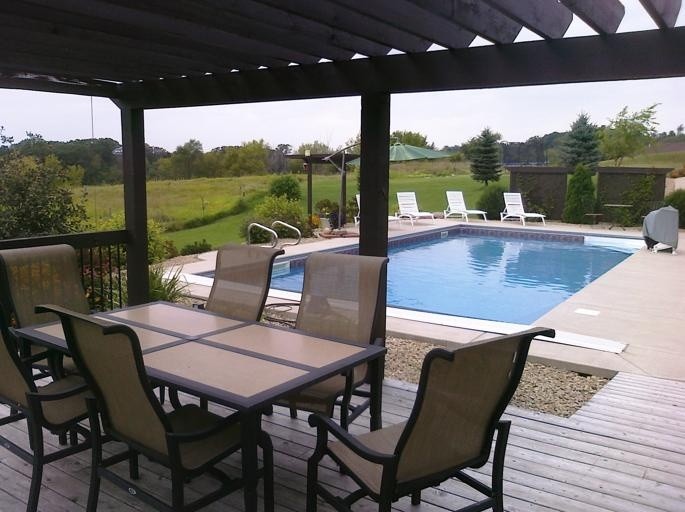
[11,300,388,512]
[604,204,633,232]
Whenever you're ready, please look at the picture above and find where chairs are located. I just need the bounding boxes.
[305,327,556,511]
[270,251,390,477]
[167,245,285,417]
[2,255,140,511]
[394,191,435,227]
[353,194,361,224]
[444,191,488,224]
[501,191,546,227]
[32,302,276,511]
[1,245,93,448]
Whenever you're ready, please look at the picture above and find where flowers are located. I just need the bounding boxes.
[319,206,336,218]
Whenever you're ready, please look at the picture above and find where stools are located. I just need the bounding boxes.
[586,213,603,229]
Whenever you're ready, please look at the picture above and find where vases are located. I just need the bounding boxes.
[319,216,331,229]
